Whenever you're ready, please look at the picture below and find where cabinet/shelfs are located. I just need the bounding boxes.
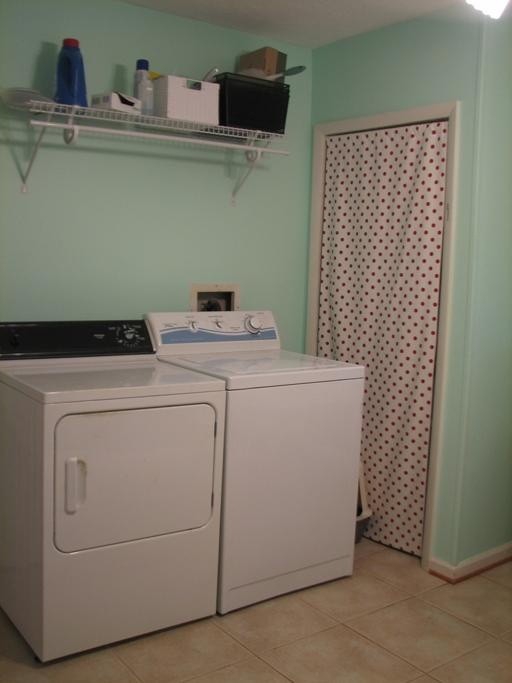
[21,96,291,201]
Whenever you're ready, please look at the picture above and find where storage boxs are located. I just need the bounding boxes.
[235,46,287,83]
[161,73,223,127]
[220,70,292,136]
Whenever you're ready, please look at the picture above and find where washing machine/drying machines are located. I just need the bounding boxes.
[0,320,226,665]
[141,309,368,616]
